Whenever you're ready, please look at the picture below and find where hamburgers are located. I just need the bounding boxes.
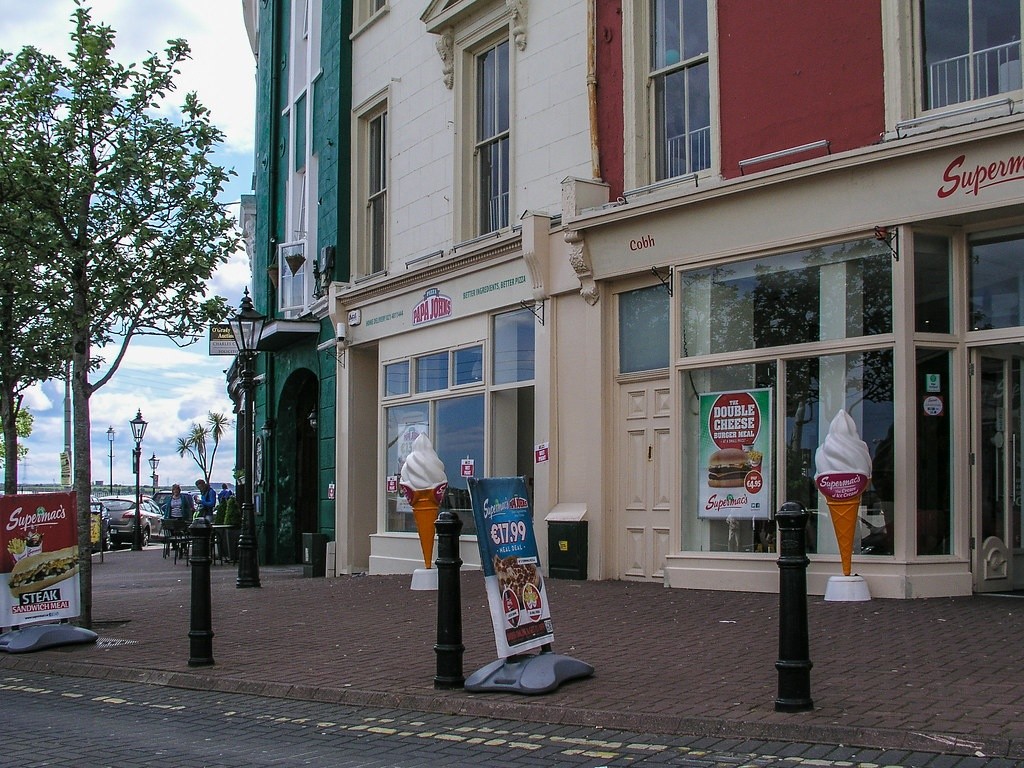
[708,448,752,487]
[8,545,81,597]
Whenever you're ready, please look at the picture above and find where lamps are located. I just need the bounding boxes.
[307,403,317,433]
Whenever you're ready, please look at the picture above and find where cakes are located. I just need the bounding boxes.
[498,556,540,599]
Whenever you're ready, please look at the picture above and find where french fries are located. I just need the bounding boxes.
[7,538,27,554]
[748,452,761,461]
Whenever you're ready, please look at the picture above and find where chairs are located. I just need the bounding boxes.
[160,519,225,566]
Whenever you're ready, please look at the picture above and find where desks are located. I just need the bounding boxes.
[213,524,235,559]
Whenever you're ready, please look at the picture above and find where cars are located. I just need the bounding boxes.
[89,495,113,551]
[151,489,204,512]
[96,494,167,546]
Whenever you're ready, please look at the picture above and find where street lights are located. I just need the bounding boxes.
[224,285,267,593]
[128,408,149,552]
[106,425,116,495]
[149,453,161,496]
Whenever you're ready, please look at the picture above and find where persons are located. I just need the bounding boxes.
[164,484,191,550]
[217,483,233,503]
[196,479,216,517]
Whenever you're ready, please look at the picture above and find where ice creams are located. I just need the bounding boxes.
[399,432,447,568]
[814,408,873,576]
